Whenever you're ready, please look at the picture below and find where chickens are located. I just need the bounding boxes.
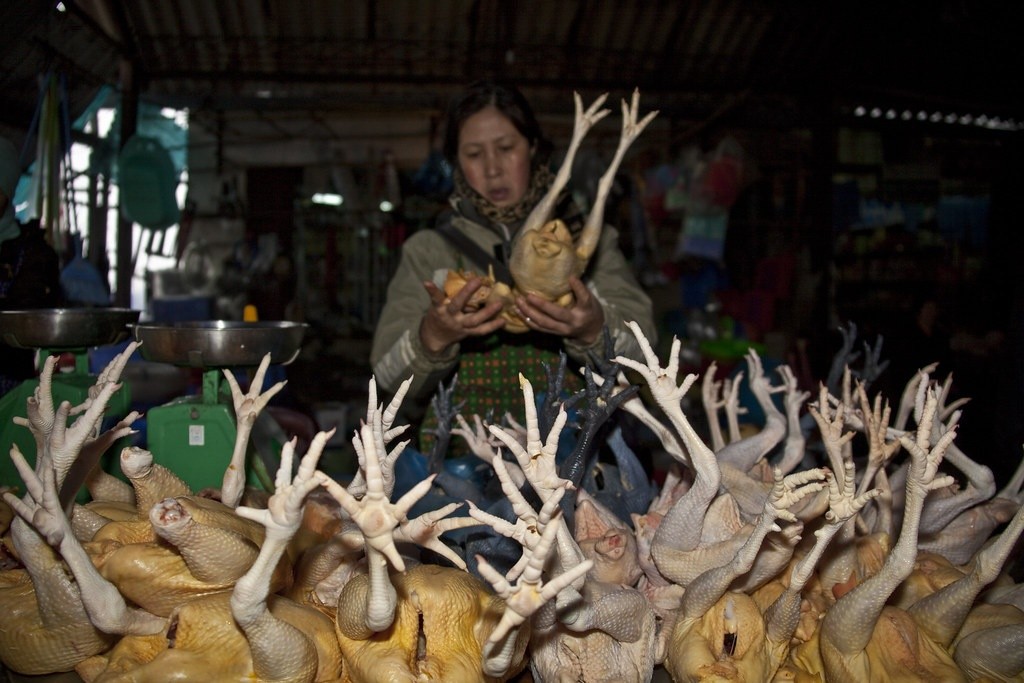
[490,87,660,335]
[0,340,1024,683]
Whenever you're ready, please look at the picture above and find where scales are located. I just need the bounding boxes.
[1,304,142,512]
[127,321,310,498]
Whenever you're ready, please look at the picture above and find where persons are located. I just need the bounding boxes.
[58,231,108,305]
[371,88,657,461]
[0,138,68,399]
[217,254,299,402]
[637,213,724,340]
[787,244,1009,464]
